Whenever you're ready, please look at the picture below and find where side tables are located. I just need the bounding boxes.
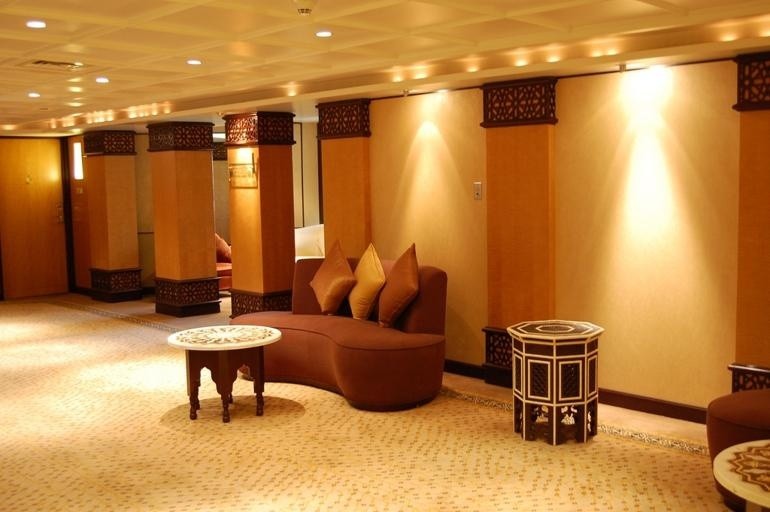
[506,319,606,445]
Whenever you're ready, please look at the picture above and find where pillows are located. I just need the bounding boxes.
[349,242,387,319]
[310,240,357,315]
[379,244,419,328]
[216,233,232,263]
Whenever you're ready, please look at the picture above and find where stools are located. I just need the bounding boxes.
[705,390,770,509]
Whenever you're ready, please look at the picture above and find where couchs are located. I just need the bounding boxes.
[231,256,450,411]
